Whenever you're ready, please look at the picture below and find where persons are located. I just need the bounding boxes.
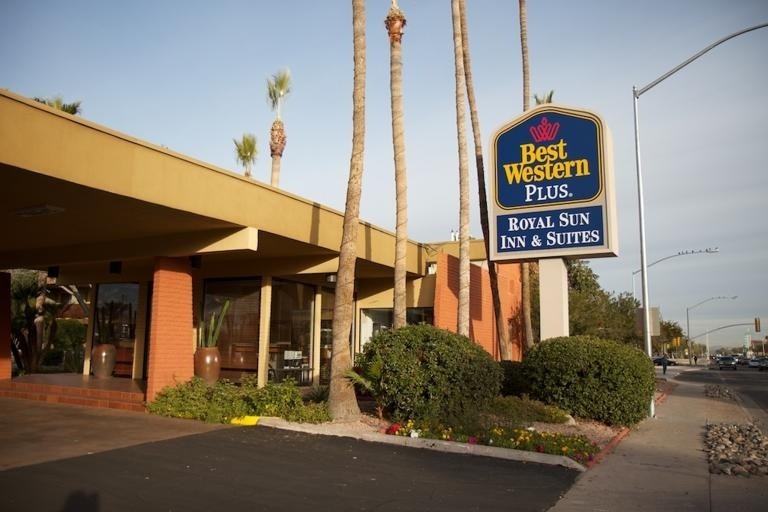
[692,354,699,366]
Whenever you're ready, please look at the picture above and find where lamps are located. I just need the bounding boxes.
[188,255,201,269]
[47,266,58,278]
[109,260,121,274]
[326,273,337,285]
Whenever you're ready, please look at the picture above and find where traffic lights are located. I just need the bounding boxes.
[756,319,761,333]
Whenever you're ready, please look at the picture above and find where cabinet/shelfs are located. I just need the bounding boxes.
[290,309,351,384]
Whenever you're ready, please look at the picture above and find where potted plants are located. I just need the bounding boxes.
[91,299,128,379]
[193,300,230,389]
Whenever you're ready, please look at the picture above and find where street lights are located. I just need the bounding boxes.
[686,295,737,339]
[632,248,719,307]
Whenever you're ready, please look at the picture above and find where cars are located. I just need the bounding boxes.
[654,357,678,366]
[711,355,768,370]
